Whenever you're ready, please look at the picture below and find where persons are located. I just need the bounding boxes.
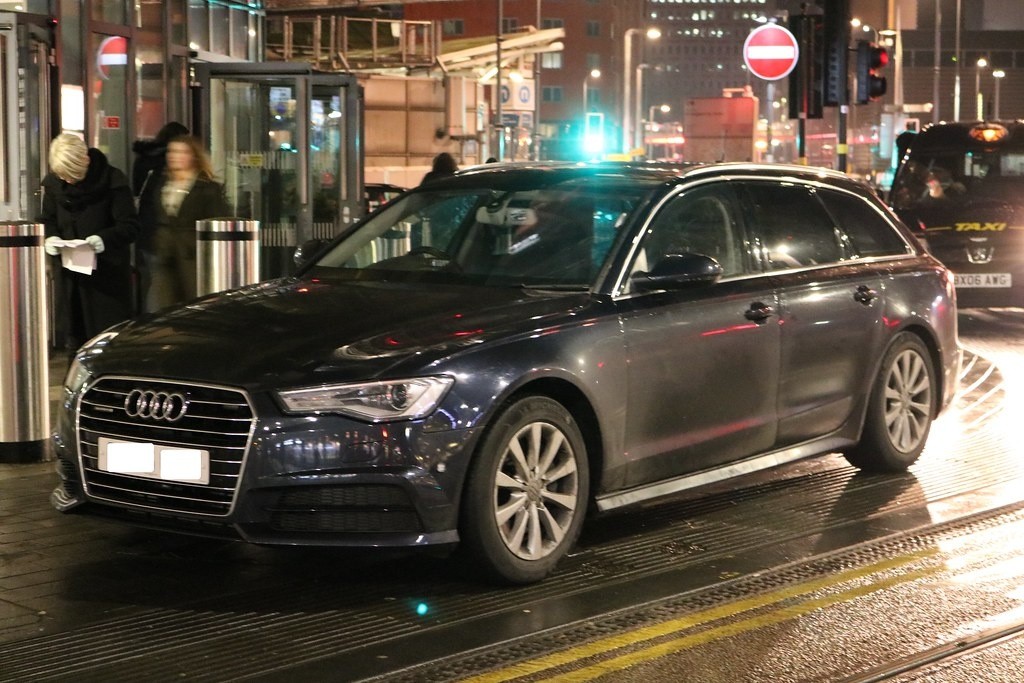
[40,133,138,387]
[895,121,918,165]
[421,152,498,185]
[133,121,235,314]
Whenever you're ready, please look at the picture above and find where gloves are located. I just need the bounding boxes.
[45,236,63,256]
[86,235,105,254]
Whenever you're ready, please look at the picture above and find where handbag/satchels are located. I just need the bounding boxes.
[133,195,141,216]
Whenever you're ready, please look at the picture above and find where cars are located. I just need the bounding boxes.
[887,116,1024,313]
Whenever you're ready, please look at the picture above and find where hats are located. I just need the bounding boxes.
[433,153,459,174]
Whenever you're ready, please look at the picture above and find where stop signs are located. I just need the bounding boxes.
[740,23,800,81]
[94,34,126,81]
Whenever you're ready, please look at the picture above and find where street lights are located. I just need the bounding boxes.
[620,27,663,159]
[648,105,670,162]
[632,64,663,161]
[880,27,904,111]
[581,68,600,161]
[974,56,988,123]
[990,68,1005,119]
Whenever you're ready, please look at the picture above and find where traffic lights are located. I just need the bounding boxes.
[856,36,890,106]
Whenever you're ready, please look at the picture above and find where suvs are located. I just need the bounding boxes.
[52,161,961,585]
